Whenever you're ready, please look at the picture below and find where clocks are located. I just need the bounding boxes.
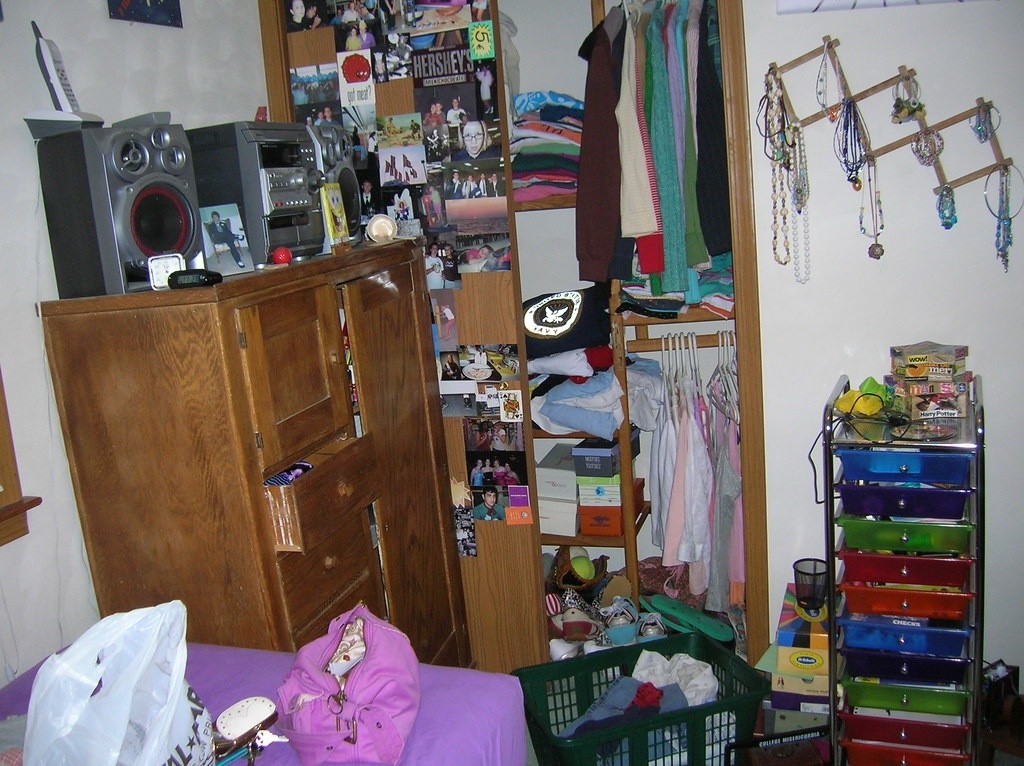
[147,253,187,291]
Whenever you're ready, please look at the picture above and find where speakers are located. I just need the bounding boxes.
[306,125,364,242]
[37,124,208,301]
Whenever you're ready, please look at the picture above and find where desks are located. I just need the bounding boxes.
[1,640,530,766]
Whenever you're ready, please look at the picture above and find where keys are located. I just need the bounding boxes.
[247,729,290,766]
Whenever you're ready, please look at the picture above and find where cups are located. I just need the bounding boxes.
[368,214,399,243]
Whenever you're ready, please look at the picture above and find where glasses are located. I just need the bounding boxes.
[462,133,484,140]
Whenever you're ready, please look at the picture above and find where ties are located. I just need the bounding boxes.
[483,181,485,194]
[366,196,369,204]
[470,184,471,193]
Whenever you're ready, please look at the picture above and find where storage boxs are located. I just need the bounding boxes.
[535,428,644,536]
[510,632,770,766]
[752,581,845,745]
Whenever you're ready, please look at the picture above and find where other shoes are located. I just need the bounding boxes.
[238,235,244,240]
[543,544,735,663]
[238,262,245,268]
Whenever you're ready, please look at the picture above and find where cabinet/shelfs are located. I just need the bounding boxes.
[259,1,769,676]
[824,376,993,766]
[34,235,474,672]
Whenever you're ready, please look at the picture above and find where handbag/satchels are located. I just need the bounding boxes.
[983,658,1020,732]
[275,599,420,766]
[23,600,215,766]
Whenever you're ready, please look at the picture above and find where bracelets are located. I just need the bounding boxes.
[890,75,1024,231]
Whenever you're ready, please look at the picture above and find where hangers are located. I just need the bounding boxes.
[659,330,742,428]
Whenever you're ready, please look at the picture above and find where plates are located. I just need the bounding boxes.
[460,360,470,367]
[463,363,493,381]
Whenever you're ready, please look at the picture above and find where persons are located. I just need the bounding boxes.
[208,211,245,268]
[287,0,522,521]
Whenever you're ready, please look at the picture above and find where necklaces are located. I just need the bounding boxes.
[755,41,885,284]
[995,168,1014,273]
